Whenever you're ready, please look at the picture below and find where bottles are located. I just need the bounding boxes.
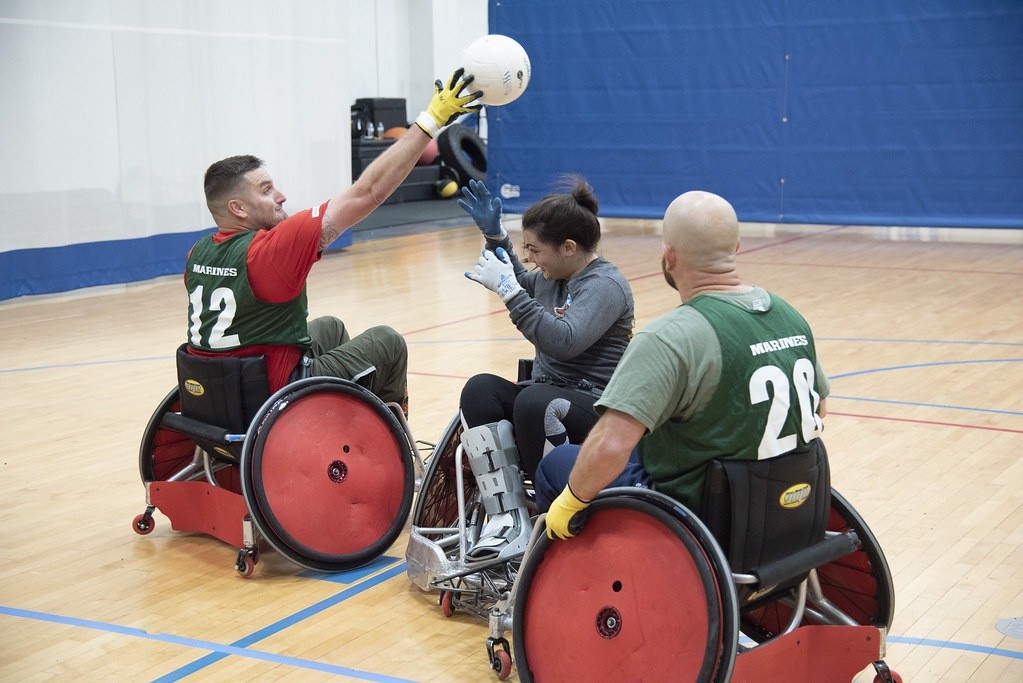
[378,122,384,140]
[366,121,371,136]
[368,123,374,136]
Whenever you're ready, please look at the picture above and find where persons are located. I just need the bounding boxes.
[457,169,634,563]
[536,190,829,541]
[183,69,482,434]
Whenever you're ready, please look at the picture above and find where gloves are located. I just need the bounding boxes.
[415,67,484,139]
[545,483,591,541]
[465,247,524,305]
[457,179,508,242]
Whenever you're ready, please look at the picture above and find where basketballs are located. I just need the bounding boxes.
[460,34,532,107]
[435,178,459,199]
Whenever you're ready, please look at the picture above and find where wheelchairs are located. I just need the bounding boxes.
[131,342,436,579]
[403,359,539,621]
[485,484,903,683]
